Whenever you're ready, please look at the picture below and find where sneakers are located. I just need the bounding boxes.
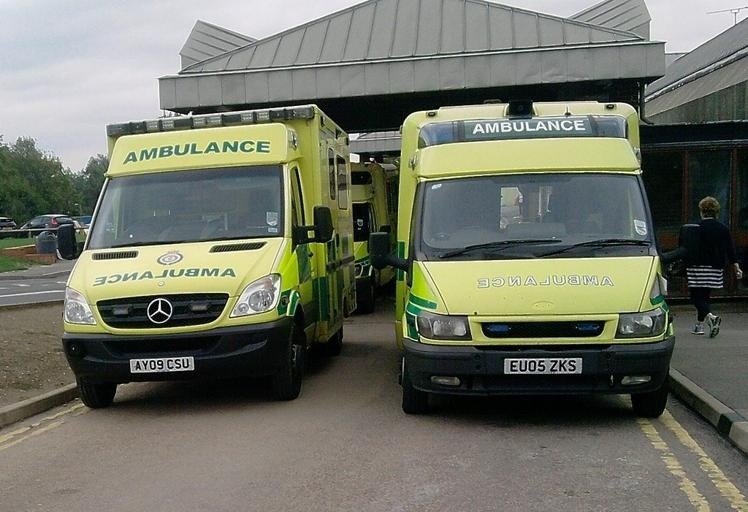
[708,315,721,338]
[691,325,704,335]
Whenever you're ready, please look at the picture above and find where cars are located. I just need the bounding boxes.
[1,213,93,240]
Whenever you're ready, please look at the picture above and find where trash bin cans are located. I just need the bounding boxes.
[35,223,77,261]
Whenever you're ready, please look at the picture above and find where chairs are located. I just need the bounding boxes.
[132,188,281,243]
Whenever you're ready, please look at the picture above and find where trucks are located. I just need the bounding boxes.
[349,161,401,314]
[369,100,674,420]
[56,103,358,410]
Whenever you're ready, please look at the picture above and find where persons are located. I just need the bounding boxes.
[685,195,744,340]
